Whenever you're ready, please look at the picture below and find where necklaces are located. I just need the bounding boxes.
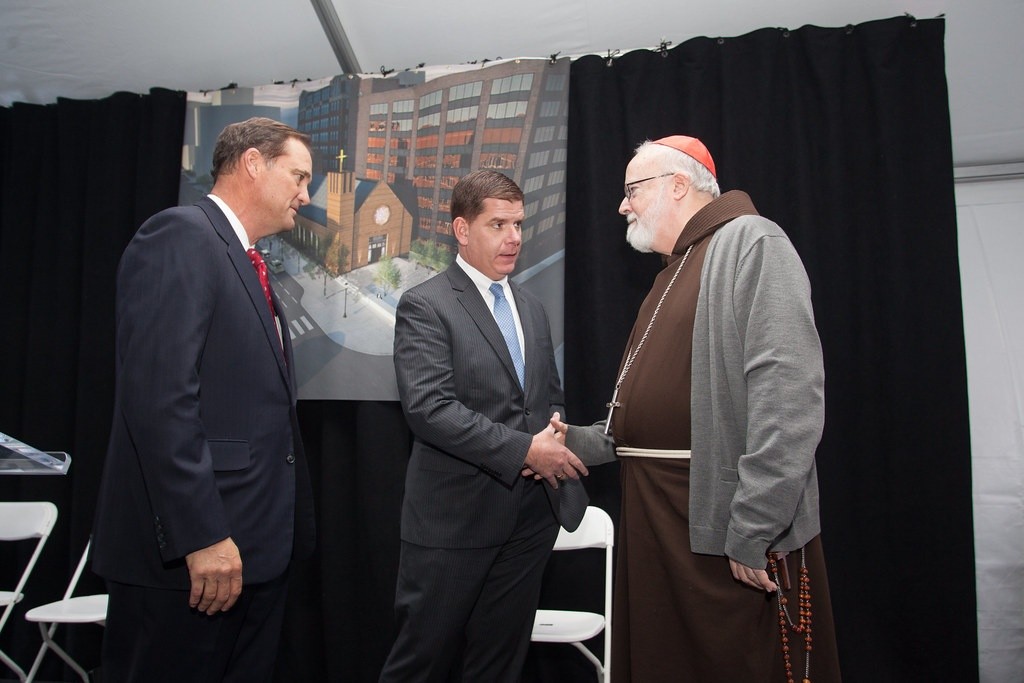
[604,245,693,434]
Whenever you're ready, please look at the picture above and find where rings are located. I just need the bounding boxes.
[557,474,563,479]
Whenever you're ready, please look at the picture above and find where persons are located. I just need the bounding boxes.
[88,116,316,683]
[524,136,840,683]
[377,171,589,683]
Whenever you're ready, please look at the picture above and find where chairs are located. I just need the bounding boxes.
[0,502,59,683]
[24,532,109,683]
[528,502,616,683]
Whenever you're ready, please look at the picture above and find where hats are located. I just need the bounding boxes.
[649,135,718,178]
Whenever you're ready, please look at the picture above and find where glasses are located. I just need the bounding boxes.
[623,174,673,200]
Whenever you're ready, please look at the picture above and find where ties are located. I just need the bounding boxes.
[489,282,527,391]
[247,248,289,365]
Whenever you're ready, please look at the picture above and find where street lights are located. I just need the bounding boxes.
[281,238,283,261]
[343,283,348,318]
